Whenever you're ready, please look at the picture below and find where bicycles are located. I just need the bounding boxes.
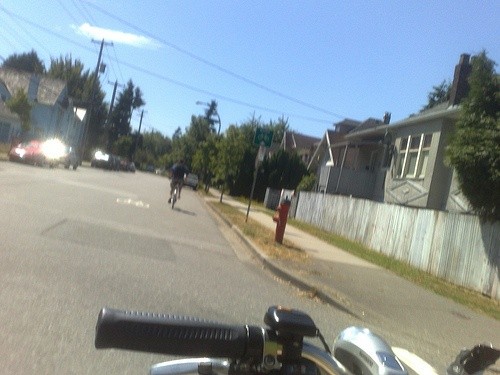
[169,175,185,209]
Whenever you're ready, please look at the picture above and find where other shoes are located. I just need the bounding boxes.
[168,198,171,203]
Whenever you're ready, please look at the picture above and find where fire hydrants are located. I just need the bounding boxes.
[272,196,291,243]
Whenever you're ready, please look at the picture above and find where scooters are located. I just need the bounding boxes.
[94,303,500,375]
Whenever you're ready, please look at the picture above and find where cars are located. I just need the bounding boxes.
[91,153,128,170]
[186,173,200,190]
[8,140,79,170]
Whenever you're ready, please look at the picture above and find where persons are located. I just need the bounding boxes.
[168,160,188,203]
